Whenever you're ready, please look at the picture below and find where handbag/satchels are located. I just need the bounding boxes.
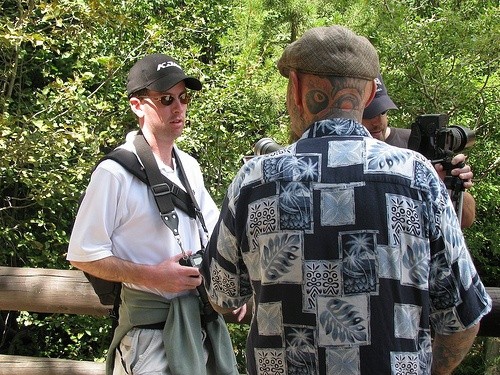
[83,271,118,305]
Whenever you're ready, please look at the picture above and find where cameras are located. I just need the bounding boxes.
[242,137,283,164]
[405,113,476,169]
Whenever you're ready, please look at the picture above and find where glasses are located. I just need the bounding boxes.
[377,110,387,116]
[137,94,191,105]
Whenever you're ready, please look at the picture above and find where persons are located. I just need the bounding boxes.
[200,24,492,375]
[66,54,239,375]
[361,74,476,228]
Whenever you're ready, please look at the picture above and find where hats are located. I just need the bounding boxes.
[362,72,399,118]
[127,53,202,96]
[277,26,379,81]
